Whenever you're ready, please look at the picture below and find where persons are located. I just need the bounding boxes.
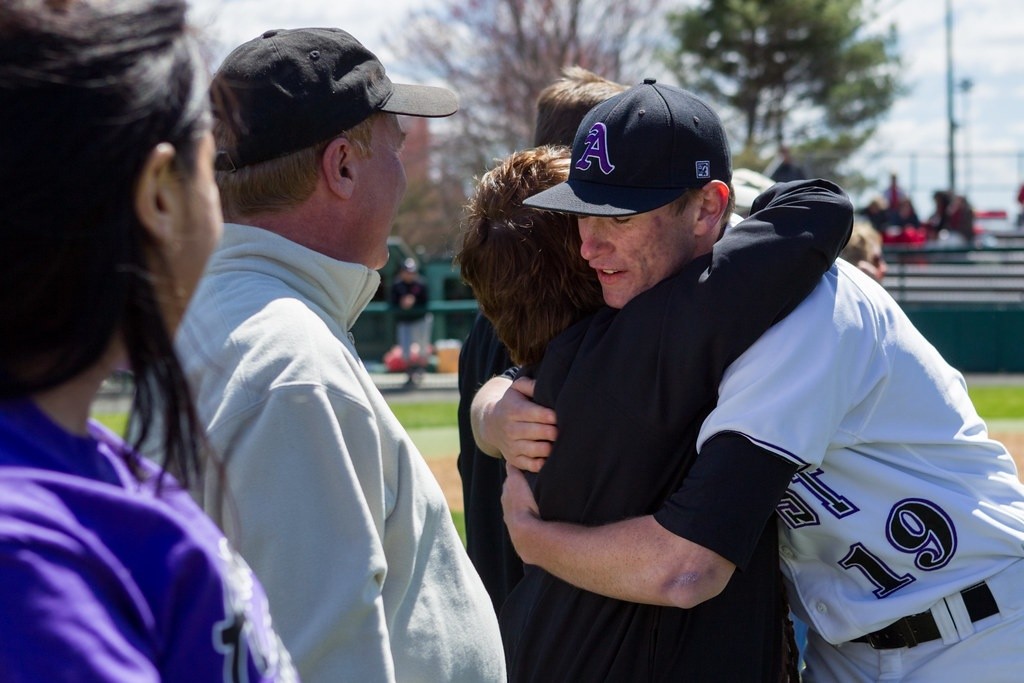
[0,1,299,683]
[457,65,1024,683]
[127,24,509,681]
[384,257,428,356]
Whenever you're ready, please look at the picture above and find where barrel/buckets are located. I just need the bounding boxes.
[436,339,462,372]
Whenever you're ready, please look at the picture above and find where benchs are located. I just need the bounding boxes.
[880,226,1024,306]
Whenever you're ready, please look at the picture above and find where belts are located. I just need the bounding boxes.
[849,580,999,650]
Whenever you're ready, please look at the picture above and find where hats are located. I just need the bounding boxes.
[208,28,458,174]
[522,77,731,217]
